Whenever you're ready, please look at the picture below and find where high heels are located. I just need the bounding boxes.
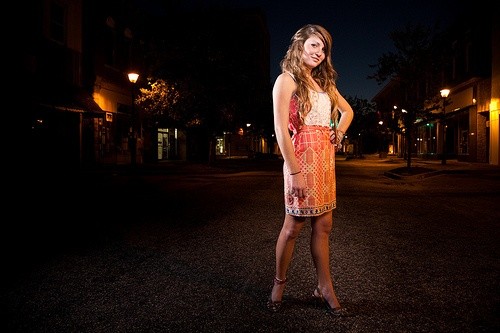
[312,288,349,318]
[265,276,288,314]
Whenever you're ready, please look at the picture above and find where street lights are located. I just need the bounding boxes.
[440,88,451,166]
[126,70,140,164]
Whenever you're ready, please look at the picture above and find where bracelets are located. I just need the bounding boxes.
[289,171,302,176]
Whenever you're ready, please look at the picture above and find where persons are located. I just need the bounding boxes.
[268,25,354,316]
